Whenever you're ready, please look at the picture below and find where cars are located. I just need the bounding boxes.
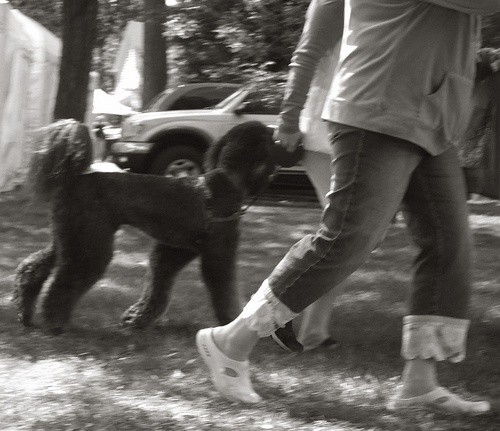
[101,83,243,147]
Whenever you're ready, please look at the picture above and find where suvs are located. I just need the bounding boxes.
[111,79,315,184]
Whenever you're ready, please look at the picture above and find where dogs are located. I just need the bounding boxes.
[10,118,306,340]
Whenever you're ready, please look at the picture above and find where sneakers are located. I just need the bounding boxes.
[319,339,341,350]
[271,320,303,353]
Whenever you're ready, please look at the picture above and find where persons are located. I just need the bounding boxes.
[195,0,500,421]
[270,0,354,354]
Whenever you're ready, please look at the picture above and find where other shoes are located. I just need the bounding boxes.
[196,327,259,403]
[388,386,489,417]
